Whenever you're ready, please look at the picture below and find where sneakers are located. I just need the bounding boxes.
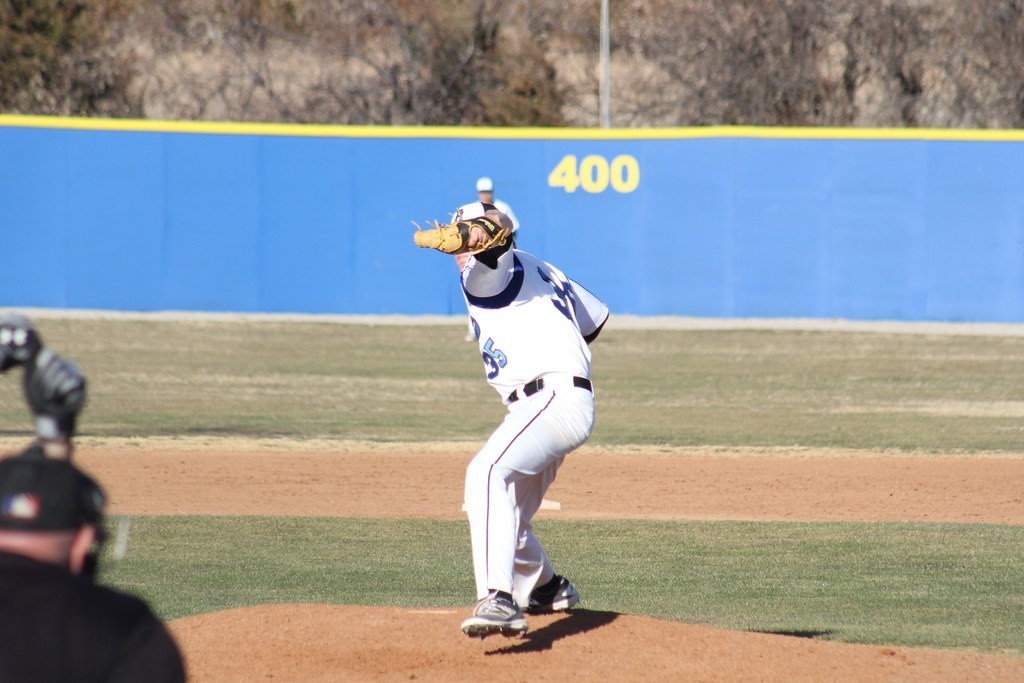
[520,574,580,614]
[461,589,529,640]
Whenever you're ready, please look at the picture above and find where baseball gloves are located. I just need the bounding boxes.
[411,207,509,255]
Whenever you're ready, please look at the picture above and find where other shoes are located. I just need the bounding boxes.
[465,333,477,342]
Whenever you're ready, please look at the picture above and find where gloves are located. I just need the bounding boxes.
[24,348,86,440]
[0,321,40,371]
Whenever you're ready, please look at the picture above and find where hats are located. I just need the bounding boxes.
[0,454,102,530]
[451,203,498,223]
[476,176,493,192]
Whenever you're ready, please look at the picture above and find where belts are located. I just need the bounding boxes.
[509,377,593,402]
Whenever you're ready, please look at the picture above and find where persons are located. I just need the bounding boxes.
[0,311,88,463]
[0,447,187,683]
[416,202,609,641]
[451,176,520,342]
